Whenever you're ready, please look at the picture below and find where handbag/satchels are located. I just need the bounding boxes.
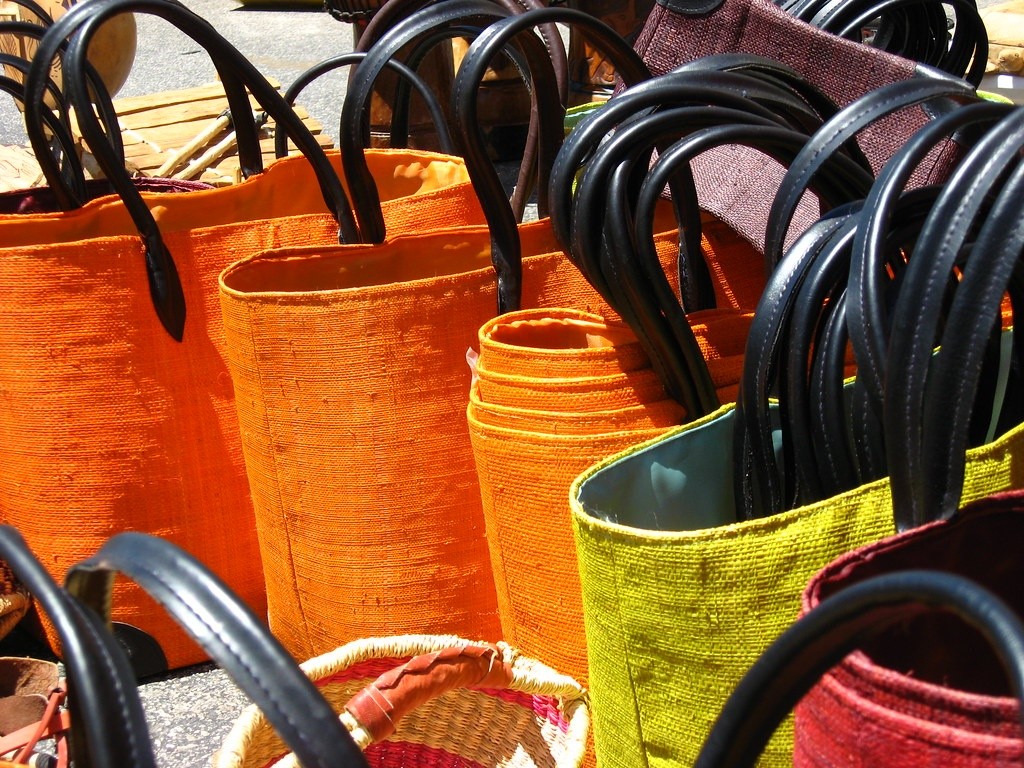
[219,6,1024,768]
[0,657,71,768]
[0,0,492,684]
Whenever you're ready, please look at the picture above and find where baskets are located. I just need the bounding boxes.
[216,634,589,768]
